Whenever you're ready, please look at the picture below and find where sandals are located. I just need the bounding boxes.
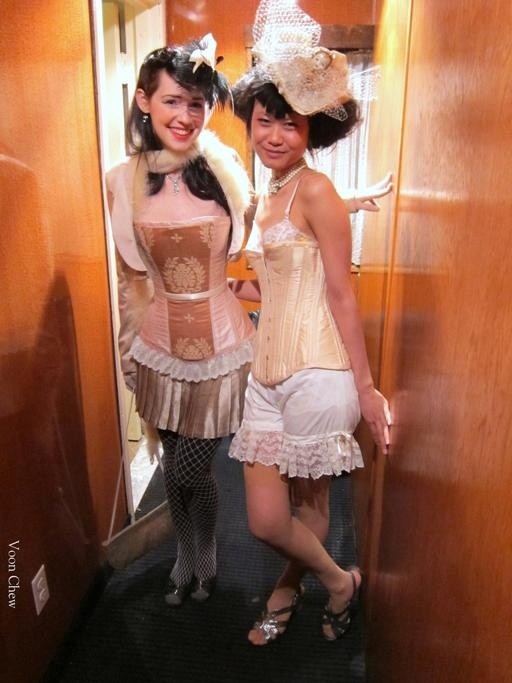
[248,581,306,646]
[321,564,366,644]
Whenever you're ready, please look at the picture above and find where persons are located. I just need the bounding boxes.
[106,46,393,645]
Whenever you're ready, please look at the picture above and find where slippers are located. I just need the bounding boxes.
[188,560,217,604]
[164,570,193,608]
